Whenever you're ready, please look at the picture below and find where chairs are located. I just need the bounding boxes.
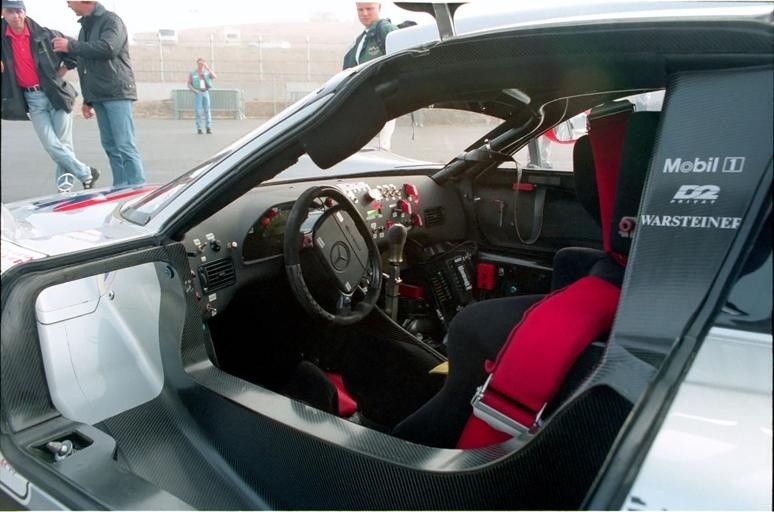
[380,108,661,451]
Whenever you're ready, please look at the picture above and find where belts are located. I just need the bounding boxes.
[21,85,41,93]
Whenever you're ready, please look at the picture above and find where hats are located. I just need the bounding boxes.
[2,0,25,10]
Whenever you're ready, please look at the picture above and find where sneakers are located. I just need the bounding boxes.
[82,166,98,189]
[197,129,212,135]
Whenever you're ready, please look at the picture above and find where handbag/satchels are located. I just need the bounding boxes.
[375,17,417,52]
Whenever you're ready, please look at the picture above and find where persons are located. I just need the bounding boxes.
[1,1,100,193]
[343,3,402,150]
[187,57,217,135]
[51,1,146,185]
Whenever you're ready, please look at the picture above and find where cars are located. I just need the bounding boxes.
[0,2,774,512]
